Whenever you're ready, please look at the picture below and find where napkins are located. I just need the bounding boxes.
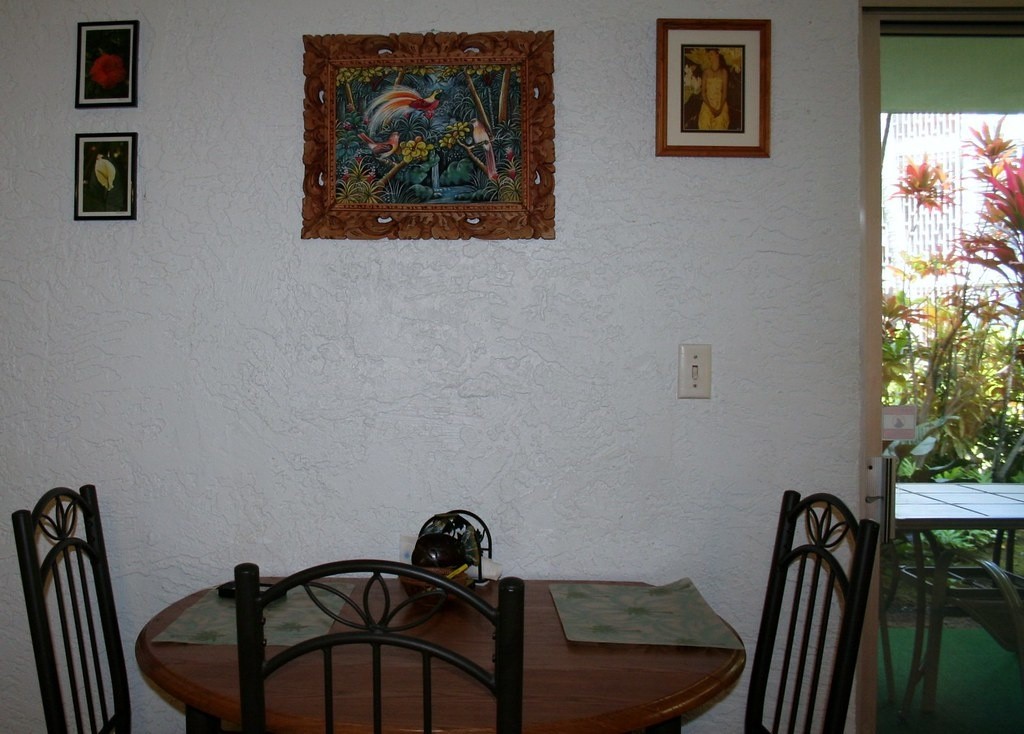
[151,582,355,646]
[549,577,747,649]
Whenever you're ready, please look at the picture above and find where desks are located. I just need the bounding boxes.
[891,483,1024,721]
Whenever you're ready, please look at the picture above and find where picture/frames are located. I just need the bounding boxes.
[302,30,556,240]
[656,18,772,157]
[73,132,138,221]
[75,20,140,108]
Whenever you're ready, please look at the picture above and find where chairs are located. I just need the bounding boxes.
[746,490,881,734]
[11,484,131,734]
[233,564,524,734]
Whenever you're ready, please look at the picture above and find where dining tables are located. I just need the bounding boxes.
[136,577,746,734]
